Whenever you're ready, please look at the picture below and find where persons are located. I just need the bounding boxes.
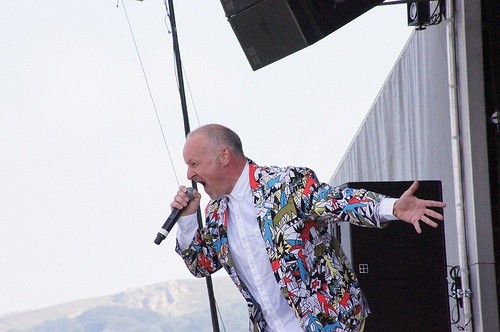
[170,124,446,332]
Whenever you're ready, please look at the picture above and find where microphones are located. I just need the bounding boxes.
[154,187,195,245]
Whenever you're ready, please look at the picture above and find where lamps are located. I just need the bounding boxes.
[407,0,430,26]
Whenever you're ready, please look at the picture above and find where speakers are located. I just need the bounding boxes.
[220,0,385,72]
[330,180,451,332]
